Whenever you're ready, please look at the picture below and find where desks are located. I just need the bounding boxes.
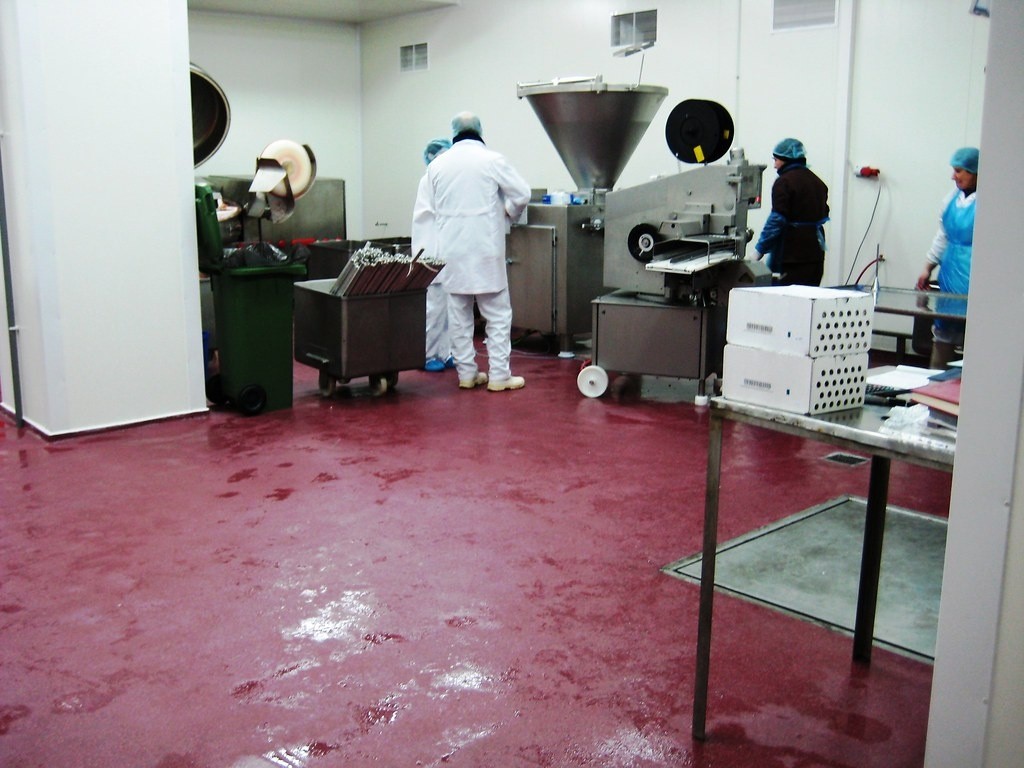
[690,365,958,744]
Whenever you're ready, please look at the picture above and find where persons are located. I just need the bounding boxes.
[425,111,533,392]
[753,137,831,287]
[916,146,979,372]
[410,139,453,373]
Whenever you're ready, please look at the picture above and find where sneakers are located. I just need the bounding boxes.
[459,372,488,389]
[486,376,525,391]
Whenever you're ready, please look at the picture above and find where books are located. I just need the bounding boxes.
[910,365,962,442]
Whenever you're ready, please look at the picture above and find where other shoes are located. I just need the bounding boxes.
[447,356,453,369]
[425,356,445,371]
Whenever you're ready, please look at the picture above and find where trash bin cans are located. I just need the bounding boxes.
[194,182,308,417]
